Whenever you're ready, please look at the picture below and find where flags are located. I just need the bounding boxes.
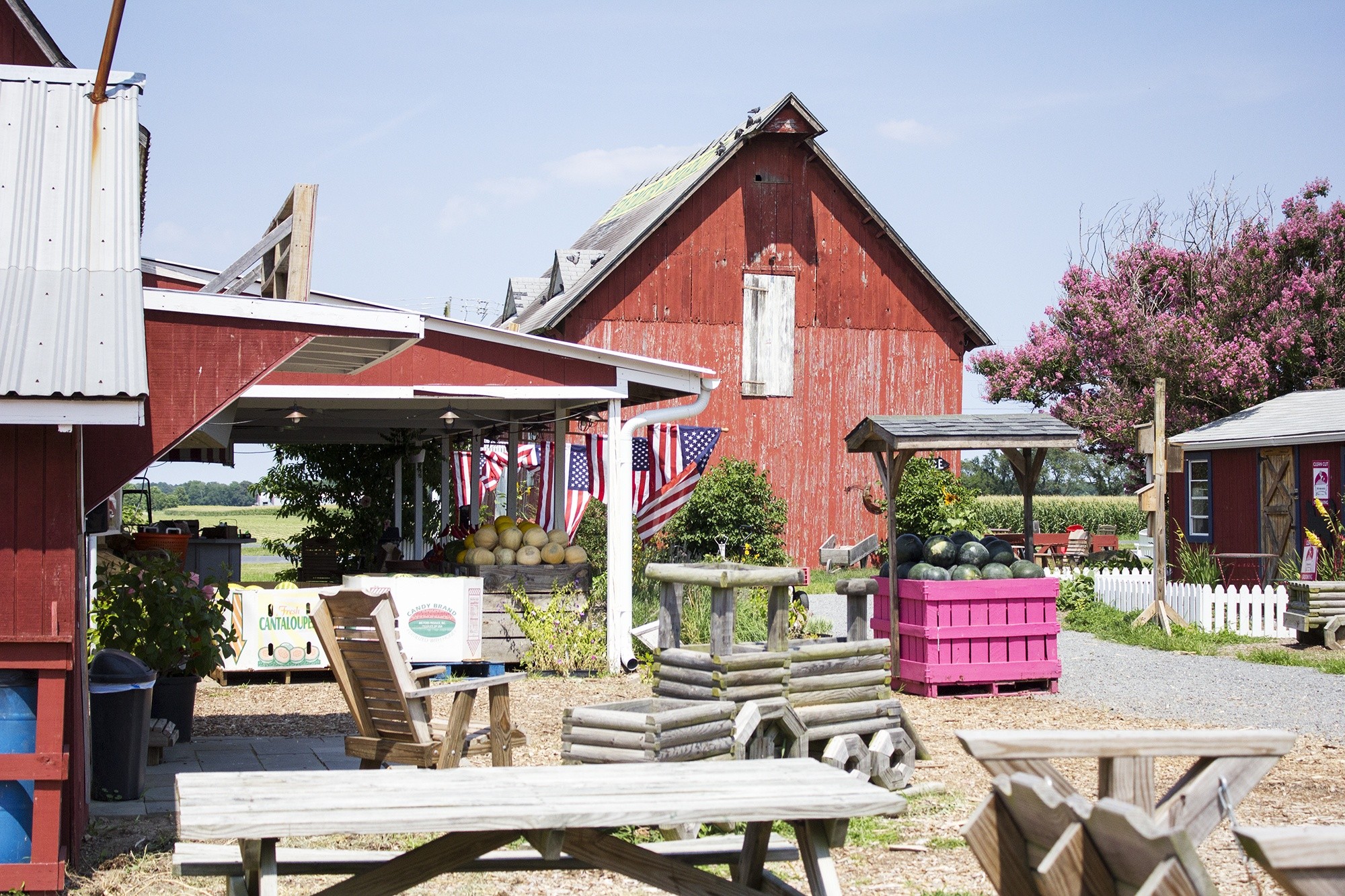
[565,443,590,549]
[632,437,648,515]
[585,431,609,506]
[633,422,722,553]
[535,440,554,534]
[479,448,508,492]
[504,443,542,471]
[451,450,472,529]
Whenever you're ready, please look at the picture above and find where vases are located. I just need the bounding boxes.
[152,672,202,743]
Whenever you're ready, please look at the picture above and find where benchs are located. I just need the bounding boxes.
[174,835,797,896]
[147,718,179,766]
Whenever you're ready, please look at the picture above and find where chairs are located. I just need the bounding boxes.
[960,772,1225,895]
[295,537,339,583]
[309,590,528,766]
[1061,524,1117,566]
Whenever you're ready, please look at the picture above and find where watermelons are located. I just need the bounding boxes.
[878,530,1046,582]
[443,540,467,563]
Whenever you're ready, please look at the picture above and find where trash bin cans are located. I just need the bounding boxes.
[86,648,158,803]
[133,531,193,572]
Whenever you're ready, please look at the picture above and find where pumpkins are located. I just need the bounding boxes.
[464,527,588,565]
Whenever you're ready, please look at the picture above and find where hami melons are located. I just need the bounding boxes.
[457,515,541,560]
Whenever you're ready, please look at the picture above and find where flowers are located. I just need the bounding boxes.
[87,549,240,679]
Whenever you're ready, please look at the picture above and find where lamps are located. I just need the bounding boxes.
[451,434,467,446]
[487,424,505,438]
[438,407,459,424]
[284,403,309,423]
[529,416,552,432]
[582,402,602,421]
[274,450,284,457]
[430,437,442,449]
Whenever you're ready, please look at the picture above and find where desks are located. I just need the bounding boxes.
[174,758,908,896]
